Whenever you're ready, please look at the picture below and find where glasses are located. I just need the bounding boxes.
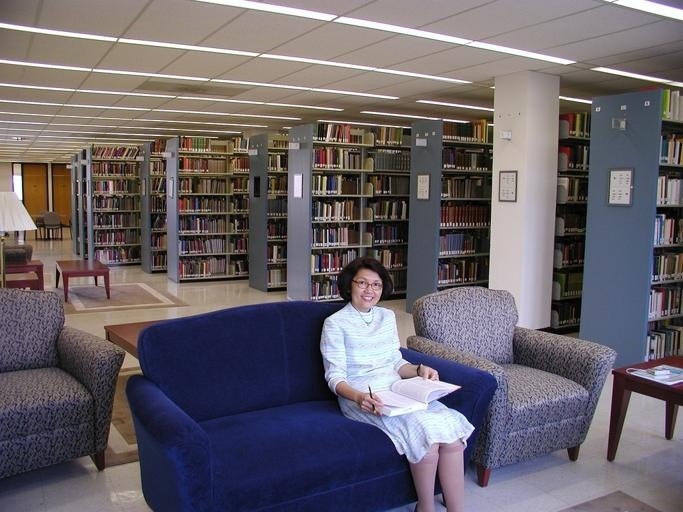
[352,278,385,291]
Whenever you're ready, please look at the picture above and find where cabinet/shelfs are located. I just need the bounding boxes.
[140,141,167,274]
[164,134,249,283]
[539,111,591,332]
[247,131,289,293]
[69,142,141,268]
[577,89,682,370]
[405,118,495,312]
[286,121,412,303]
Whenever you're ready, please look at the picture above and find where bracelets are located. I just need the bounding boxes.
[416,363,423,376]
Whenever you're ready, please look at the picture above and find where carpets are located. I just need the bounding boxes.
[560,488,661,512]
[102,367,145,468]
[49,280,189,315]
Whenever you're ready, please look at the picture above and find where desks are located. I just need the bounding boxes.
[99,320,164,355]
[607,354,682,461]
[4,259,43,291]
[54,258,110,303]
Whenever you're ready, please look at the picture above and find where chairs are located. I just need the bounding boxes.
[43,213,62,239]
[0,290,125,472]
[406,286,616,485]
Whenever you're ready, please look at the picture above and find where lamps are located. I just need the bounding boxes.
[0,191,37,287]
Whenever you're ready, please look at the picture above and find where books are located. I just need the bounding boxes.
[91,143,142,263]
[632,365,683,386]
[149,138,167,269]
[370,376,461,417]
[267,139,288,288]
[438,119,493,285]
[178,135,250,278]
[552,112,592,326]
[310,123,409,300]
[643,89,683,363]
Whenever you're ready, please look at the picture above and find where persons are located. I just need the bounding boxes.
[319,257,476,512]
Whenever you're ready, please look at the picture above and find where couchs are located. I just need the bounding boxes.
[124,297,496,512]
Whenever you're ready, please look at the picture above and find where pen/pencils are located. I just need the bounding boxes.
[368,385,376,414]
